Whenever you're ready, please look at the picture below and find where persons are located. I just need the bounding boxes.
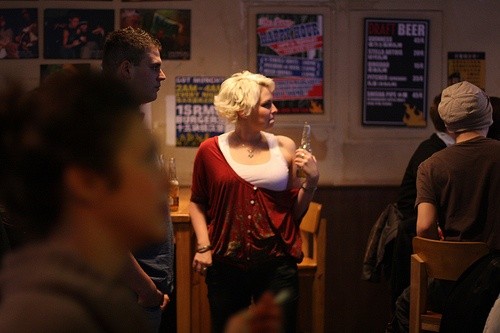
[62,17,106,59]
[189,70,320,333]
[101,27,175,333]
[122,9,142,26]
[396,80,500,333]
[0,10,38,59]
[0,72,282,333]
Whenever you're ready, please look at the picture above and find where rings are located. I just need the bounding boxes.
[302,154,304,158]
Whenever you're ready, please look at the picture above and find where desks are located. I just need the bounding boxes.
[169,200,192,333]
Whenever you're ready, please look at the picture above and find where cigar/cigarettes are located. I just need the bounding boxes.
[272,291,291,304]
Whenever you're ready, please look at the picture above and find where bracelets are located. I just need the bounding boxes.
[301,184,317,192]
[196,246,211,253]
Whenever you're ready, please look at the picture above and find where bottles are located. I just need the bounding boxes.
[296,125,312,178]
[167,157,179,212]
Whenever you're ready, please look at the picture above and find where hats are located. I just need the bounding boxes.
[438,81,493,133]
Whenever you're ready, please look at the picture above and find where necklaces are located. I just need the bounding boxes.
[235,132,260,158]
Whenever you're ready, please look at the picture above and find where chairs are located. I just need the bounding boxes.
[192,200,328,333]
[408,235,492,333]
